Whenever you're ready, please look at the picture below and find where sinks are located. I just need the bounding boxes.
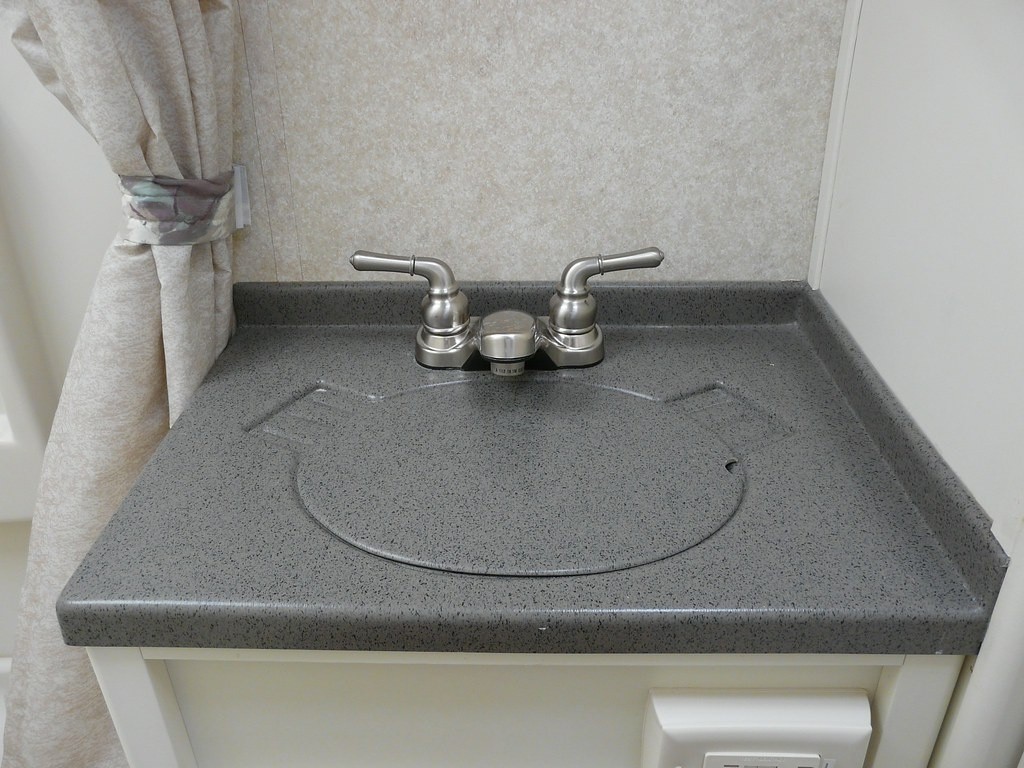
[282,372,752,583]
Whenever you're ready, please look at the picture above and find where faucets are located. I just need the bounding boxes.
[474,307,543,381]
